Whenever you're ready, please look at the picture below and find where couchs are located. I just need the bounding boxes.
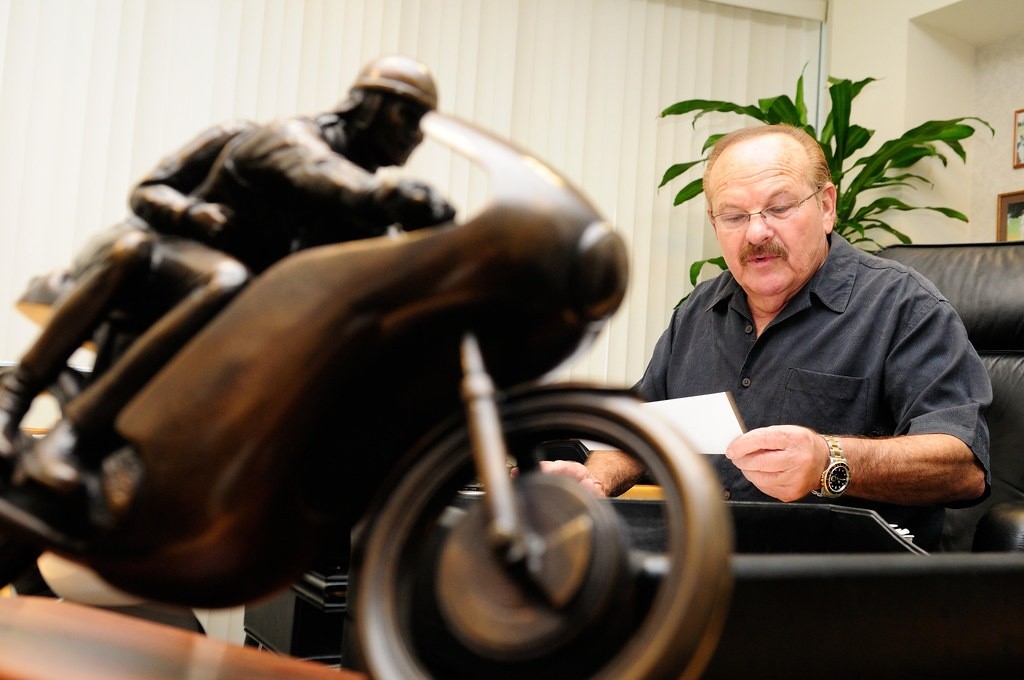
[632,239,1024,680]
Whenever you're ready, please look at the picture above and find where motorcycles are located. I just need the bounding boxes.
[1,111,733,680]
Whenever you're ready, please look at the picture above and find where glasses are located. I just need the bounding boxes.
[707,184,824,234]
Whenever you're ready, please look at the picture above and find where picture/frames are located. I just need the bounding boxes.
[1012,107,1024,169]
[996,190,1024,242]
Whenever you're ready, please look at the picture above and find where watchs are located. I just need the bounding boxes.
[812,435,851,497]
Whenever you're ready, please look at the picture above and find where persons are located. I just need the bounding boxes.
[1,56,454,487]
[509,126,993,553]
